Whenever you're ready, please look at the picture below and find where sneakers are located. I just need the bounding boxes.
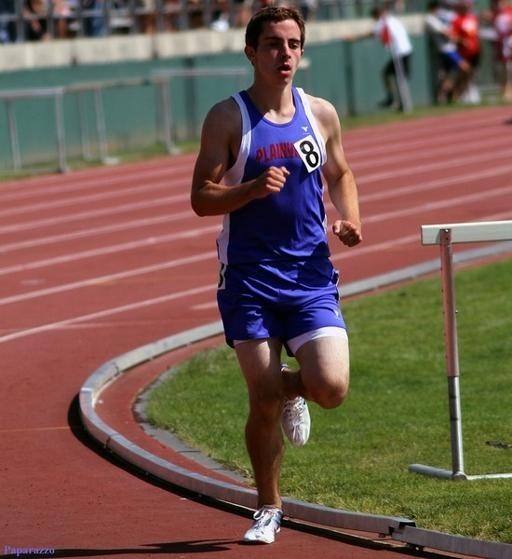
[279,363,311,448]
[243,504,283,545]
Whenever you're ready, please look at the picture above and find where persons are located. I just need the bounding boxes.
[1,0,317,44]
[371,0,511,118]
[188,5,365,545]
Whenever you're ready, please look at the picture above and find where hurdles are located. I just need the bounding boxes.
[0,67,246,176]
[408,221,512,481]
